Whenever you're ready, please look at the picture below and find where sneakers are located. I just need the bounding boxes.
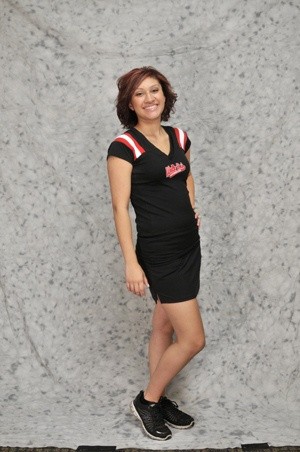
[130,390,172,440]
[158,395,195,429]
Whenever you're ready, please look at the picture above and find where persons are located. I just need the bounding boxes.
[106,66,205,441]
